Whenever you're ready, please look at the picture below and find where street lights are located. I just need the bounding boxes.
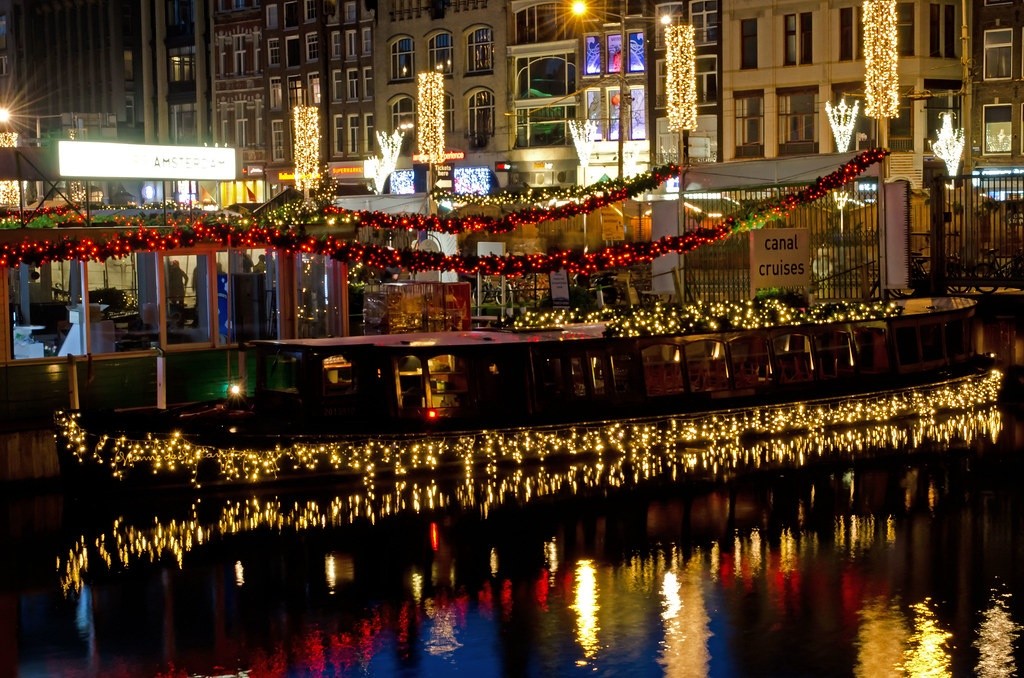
[571,0,626,183]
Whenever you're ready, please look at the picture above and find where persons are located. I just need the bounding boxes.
[169,259,188,306]
[191,262,228,306]
[243,254,265,273]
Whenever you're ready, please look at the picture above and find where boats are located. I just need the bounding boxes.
[45,294,1001,520]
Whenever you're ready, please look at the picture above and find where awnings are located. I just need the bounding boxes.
[329,191,429,226]
[647,148,883,201]
